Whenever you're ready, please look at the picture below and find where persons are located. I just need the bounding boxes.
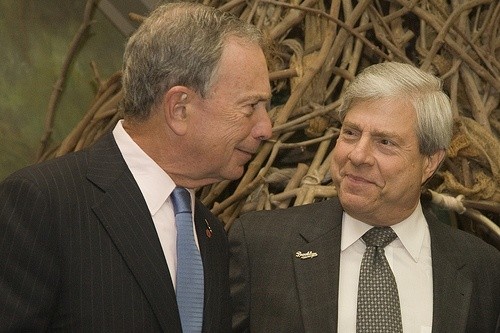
[226,62,500,333]
[0,0,273,333]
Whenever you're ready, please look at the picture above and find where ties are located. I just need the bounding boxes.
[356,225,403,333]
[170,185,204,333]
